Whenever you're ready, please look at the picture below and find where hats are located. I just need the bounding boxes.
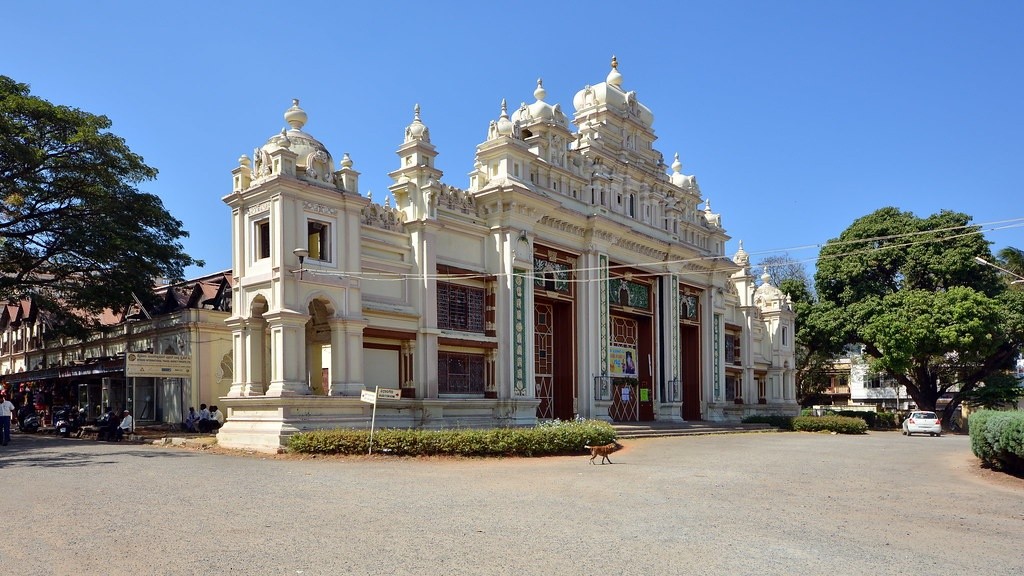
[124,409,130,413]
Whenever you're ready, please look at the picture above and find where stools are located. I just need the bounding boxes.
[122,434,134,442]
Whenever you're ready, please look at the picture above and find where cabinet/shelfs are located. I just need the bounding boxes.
[101,377,126,414]
[78,384,101,420]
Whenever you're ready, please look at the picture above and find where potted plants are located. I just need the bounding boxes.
[401,380,416,399]
[483,384,498,400]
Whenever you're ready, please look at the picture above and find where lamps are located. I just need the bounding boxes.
[288,248,309,281]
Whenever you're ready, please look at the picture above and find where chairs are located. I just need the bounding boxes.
[927,414,935,418]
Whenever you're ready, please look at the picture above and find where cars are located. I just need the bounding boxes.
[901,411,942,437]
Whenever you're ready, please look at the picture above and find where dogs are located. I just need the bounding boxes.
[585,440,625,465]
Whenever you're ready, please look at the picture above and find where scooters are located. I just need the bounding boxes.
[16,407,39,434]
[949,418,961,434]
[54,410,71,438]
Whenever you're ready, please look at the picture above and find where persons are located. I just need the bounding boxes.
[98,409,133,441]
[185,403,223,432]
[0,395,16,445]
